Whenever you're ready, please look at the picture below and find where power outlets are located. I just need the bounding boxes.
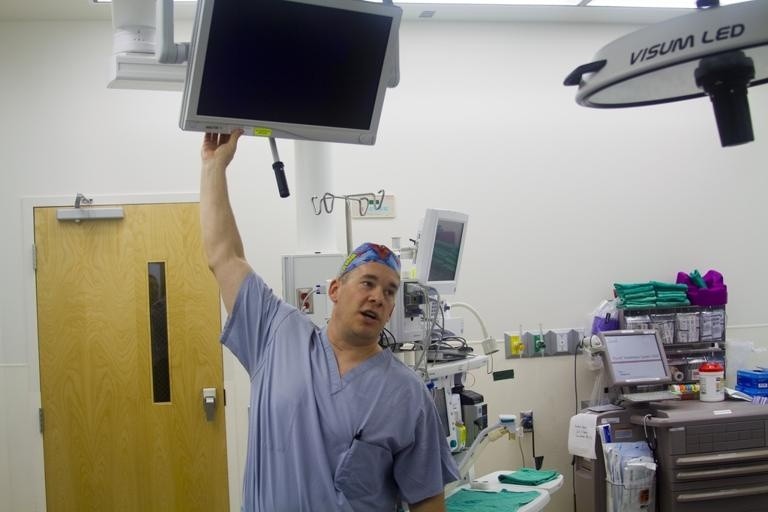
[520,411,534,432]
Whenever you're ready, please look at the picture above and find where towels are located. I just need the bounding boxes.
[612,276,690,309]
[498,468,561,486]
[685,269,706,289]
[442,488,541,512]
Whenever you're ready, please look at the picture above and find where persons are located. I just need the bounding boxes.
[200,126,464,512]
[148,273,169,402]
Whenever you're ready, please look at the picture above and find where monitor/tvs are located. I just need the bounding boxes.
[598,329,673,389]
[178,0,402,147]
[417,208,470,289]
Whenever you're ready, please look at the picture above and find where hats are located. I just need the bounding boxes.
[338,242,401,277]
